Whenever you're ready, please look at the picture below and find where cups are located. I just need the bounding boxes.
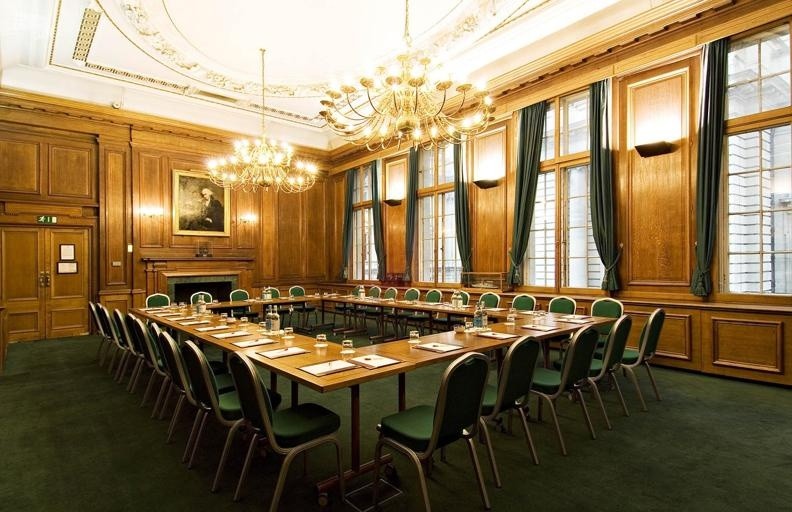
[409,330,420,343]
[509,307,518,316]
[506,315,515,325]
[315,333,327,345]
[287,288,337,300]
[466,321,473,331]
[258,321,266,332]
[339,339,354,353]
[284,327,293,336]
[172,294,248,326]
[532,310,547,320]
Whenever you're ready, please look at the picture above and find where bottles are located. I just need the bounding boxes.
[266,304,280,332]
[358,283,366,299]
[451,287,463,310]
[473,300,487,331]
[262,284,272,301]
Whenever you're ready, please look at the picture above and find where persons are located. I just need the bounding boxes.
[196,186,223,230]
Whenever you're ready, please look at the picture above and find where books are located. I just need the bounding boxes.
[229,336,280,348]
[348,353,404,368]
[298,358,362,377]
[137,293,596,341]
[413,341,466,354]
[257,345,312,359]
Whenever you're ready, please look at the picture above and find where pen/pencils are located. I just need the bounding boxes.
[493,333,501,335]
[433,345,444,347]
[365,358,378,360]
[329,362,330,368]
[532,326,539,327]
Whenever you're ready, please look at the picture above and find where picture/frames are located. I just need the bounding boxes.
[171,170,232,237]
[56,261,78,274]
[59,243,75,261]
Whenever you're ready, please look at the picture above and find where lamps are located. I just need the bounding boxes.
[205,47,318,194]
[318,1,496,152]
